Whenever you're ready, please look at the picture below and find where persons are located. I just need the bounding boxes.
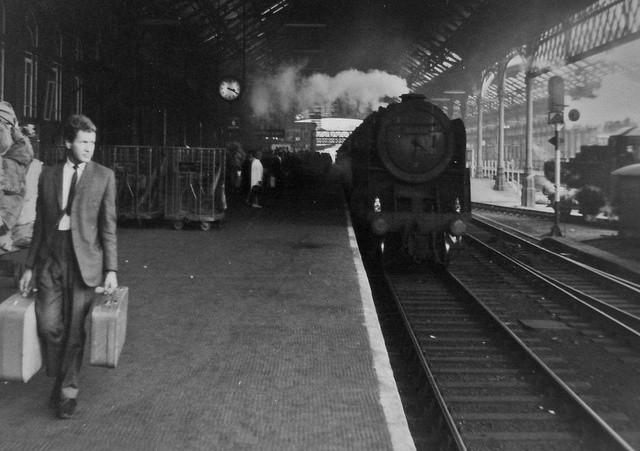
[251,150,264,208]
[18,113,119,419]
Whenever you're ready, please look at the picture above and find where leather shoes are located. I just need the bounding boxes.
[57,396,77,418]
[51,376,62,407]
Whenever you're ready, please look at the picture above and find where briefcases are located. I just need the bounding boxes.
[0,287,43,382]
[92,284,128,368]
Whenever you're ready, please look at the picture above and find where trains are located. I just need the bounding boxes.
[333,92,473,269]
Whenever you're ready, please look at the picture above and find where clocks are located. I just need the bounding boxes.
[218,75,243,101]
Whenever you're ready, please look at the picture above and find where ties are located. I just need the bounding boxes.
[50,165,78,230]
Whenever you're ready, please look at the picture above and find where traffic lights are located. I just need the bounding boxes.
[548,76,564,112]
